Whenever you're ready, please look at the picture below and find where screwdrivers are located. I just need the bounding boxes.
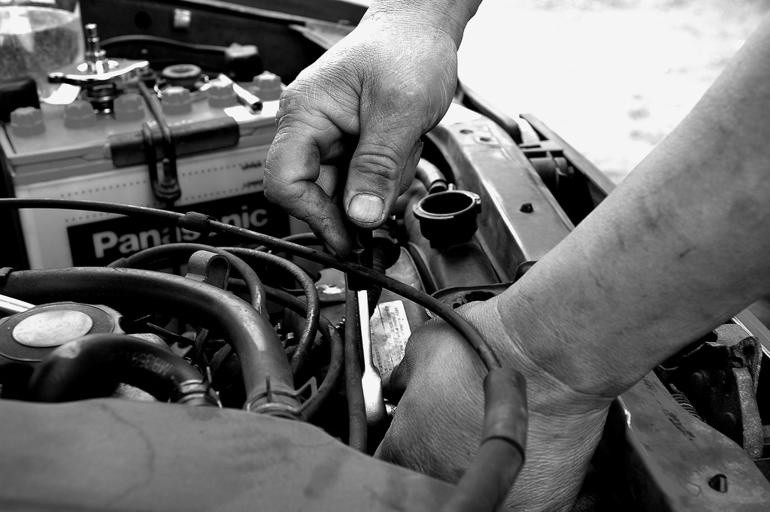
[339,131,385,426]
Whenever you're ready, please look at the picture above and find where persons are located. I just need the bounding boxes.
[261,1,769,512]
